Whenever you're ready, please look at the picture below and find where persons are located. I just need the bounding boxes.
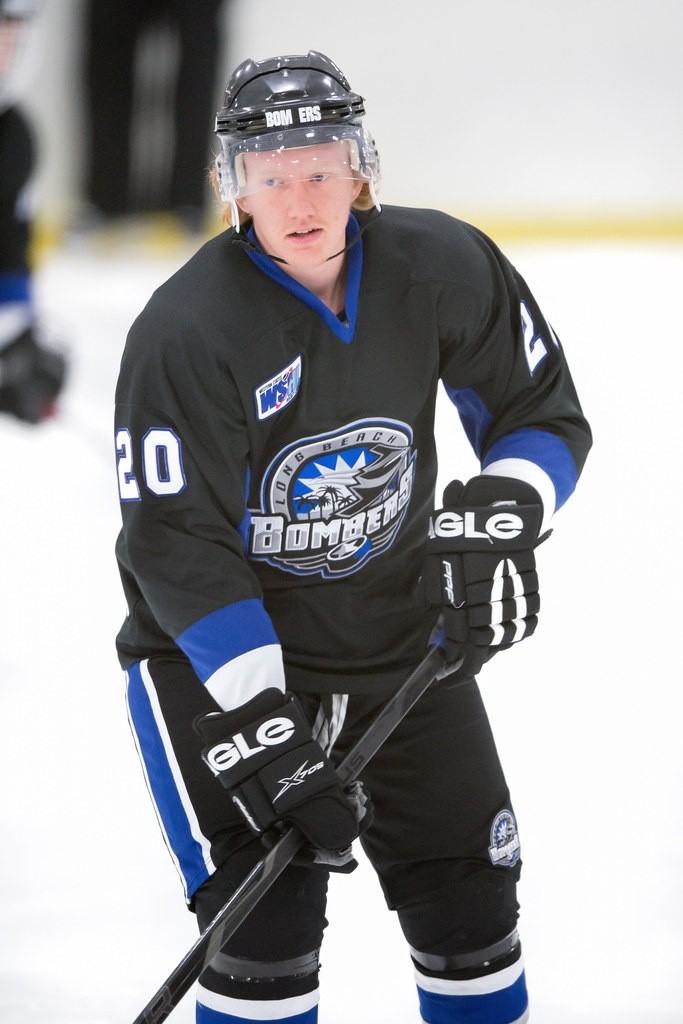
[110,50,591,1024]
[0,1,67,423]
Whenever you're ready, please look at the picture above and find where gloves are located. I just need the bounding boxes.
[193,685,374,874]
[426,474,553,690]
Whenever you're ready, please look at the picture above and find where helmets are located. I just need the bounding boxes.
[214,48,370,192]
